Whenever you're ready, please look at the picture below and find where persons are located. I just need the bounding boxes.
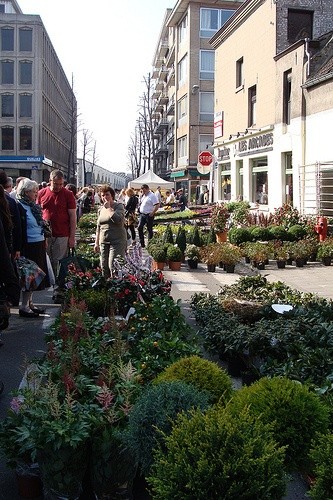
[0,169,209,345]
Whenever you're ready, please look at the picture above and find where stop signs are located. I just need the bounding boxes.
[198,151,212,166]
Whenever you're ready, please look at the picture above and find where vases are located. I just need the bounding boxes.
[12,469,43,500]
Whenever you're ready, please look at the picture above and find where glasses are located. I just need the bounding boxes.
[4,185,11,189]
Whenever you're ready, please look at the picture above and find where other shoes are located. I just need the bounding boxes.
[19,309,39,318]
[32,309,44,313]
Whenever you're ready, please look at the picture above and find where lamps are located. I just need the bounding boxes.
[229,128,261,140]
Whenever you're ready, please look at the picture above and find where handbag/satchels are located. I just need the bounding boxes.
[17,256,46,292]
[57,247,92,288]
[46,252,55,285]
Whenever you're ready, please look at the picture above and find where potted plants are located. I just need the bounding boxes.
[187,277,333,394]
[148,201,333,272]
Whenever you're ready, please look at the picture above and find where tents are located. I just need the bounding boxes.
[128,170,174,190]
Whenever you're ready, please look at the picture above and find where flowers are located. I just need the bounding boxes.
[16,254,46,293]
[0,292,109,476]
